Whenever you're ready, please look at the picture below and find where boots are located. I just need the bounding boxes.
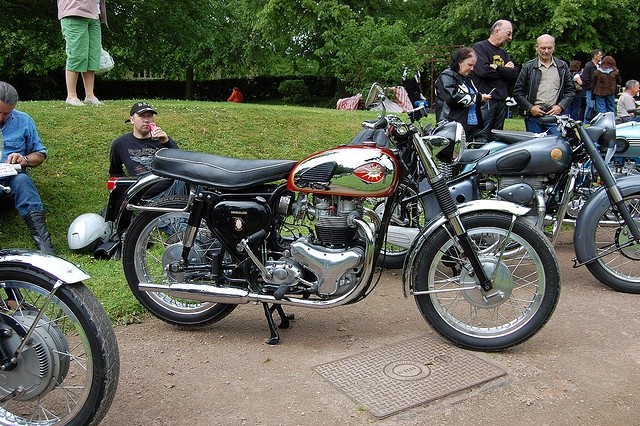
[24,210,56,256]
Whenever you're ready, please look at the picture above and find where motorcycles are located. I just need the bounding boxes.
[567,118,638,221]
[460,107,639,252]
[0,183,120,425]
[104,81,561,352]
[354,111,640,294]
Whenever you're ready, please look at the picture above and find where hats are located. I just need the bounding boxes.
[125,101,157,123]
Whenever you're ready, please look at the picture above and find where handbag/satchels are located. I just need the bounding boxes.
[99,47,114,75]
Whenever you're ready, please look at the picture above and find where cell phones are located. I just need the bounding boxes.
[489,88,497,95]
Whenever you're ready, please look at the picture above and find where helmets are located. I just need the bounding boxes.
[68,212,111,250]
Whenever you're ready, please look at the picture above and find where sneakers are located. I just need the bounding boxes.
[67,97,84,107]
[84,97,104,104]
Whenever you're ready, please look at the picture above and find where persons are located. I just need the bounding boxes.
[469,19,518,133]
[617,78,640,122]
[512,32,576,137]
[56,0,110,106]
[0,79,55,257]
[581,48,603,124]
[428,45,492,140]
[566,59,583,119]
[591,55,622,115]
[108,102,219,242]
[225,87,244,104]
